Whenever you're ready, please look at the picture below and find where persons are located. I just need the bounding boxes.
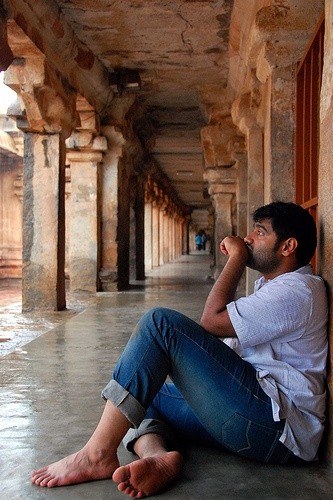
[195,231,208,250]
[29,200,329,499]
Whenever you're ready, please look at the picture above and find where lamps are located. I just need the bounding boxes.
[109,69,141,97]
